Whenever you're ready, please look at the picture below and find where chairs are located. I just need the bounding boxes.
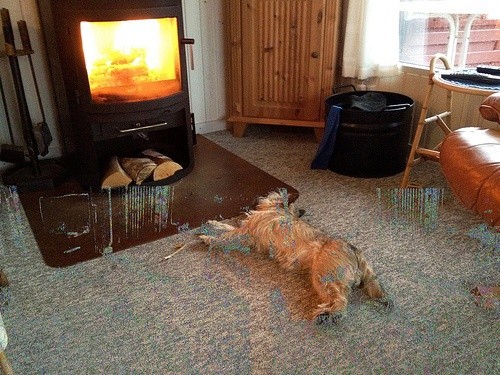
[440,92,499,232]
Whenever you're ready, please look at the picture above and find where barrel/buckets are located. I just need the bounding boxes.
[324,82,414,178]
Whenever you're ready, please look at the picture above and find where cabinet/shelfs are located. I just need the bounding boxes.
[222,0,342,144]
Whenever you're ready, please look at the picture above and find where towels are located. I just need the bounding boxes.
[309,105,343,174]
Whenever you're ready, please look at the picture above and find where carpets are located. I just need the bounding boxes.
[8,133,301,274]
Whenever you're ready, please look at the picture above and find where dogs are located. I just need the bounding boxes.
[195,188,386,326]
